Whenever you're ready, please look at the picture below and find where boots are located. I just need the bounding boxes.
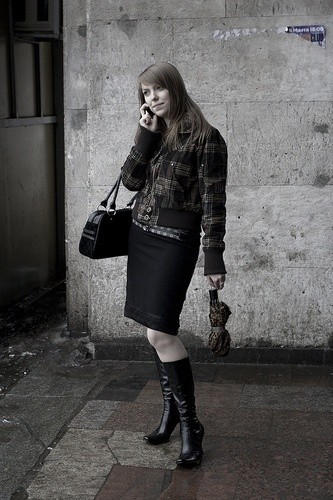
[160,356,204,466]
[142,346,181,445]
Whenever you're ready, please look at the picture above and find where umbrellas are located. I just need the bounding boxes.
[208,288,233,357]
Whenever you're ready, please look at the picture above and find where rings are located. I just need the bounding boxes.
[219,281,224,286]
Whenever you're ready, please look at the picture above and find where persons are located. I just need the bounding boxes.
[119,60,228,468]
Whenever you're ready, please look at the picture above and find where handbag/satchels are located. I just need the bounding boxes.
[79,206,132,259]
